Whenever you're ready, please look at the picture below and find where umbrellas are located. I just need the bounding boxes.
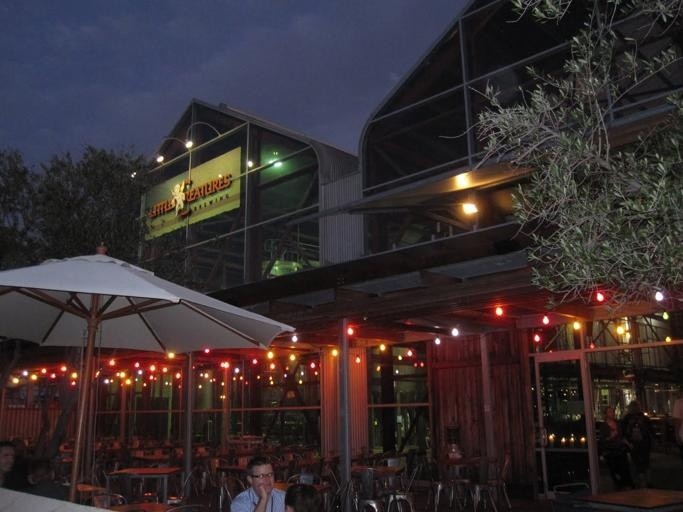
[0,240,297,503]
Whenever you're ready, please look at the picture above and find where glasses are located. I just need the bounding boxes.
[251,472,274,480]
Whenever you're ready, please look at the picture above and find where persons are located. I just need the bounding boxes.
[284,483,324,512]
[597,406,637,492]
[230,457,287,512]
[621,400,658,489]
[0,440,32,493]
[20,456,69,502]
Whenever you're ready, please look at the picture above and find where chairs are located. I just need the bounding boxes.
[59,433,514,512]
